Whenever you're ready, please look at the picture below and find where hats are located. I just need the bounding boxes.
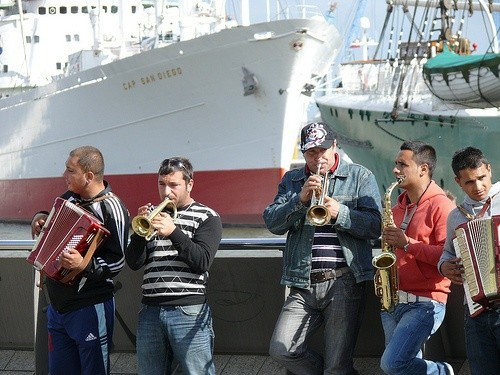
[301,121,334,154]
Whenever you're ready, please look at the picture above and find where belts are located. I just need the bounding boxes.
[311,266,350,283]
[397,290,431,303]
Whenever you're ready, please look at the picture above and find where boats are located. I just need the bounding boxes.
[0,0,500,231]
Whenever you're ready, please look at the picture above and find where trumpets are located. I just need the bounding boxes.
[131,196,178,240]
[306,164,331,226]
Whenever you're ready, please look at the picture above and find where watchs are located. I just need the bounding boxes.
[404,239,410,252]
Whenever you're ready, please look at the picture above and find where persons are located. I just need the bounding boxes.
[381,140,456,375]
[31,146,130,375]
[437,146,500,375]
[125,156,223,375]
[263,121,383,375]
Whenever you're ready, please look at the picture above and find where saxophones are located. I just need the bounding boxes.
[371,178,406,312]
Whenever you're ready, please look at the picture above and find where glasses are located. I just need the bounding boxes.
[160,159,190,175]
